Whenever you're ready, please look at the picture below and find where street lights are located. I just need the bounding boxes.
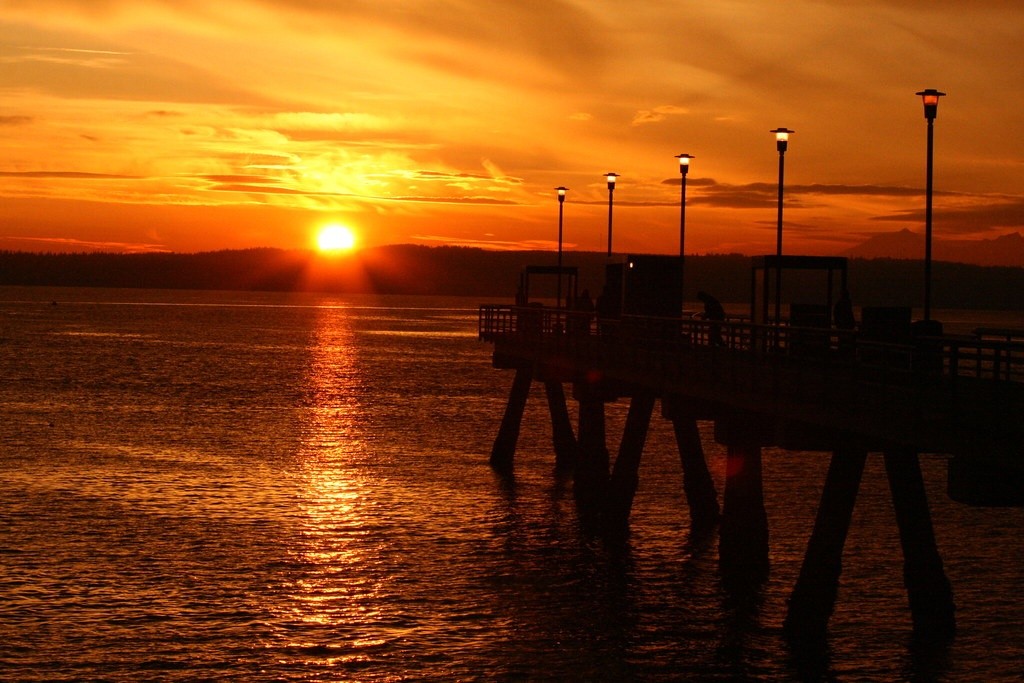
[602,172,621,320]
[915,87,946,321]
[674,153,695,338]
[555,185,570,335]
[769,127,797,352]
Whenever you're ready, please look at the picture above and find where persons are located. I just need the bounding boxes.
[596,286,608,330]
[834,289,855,329]
[582,289,594,312]
[516,287,524,321]
[696,292,724,346]
